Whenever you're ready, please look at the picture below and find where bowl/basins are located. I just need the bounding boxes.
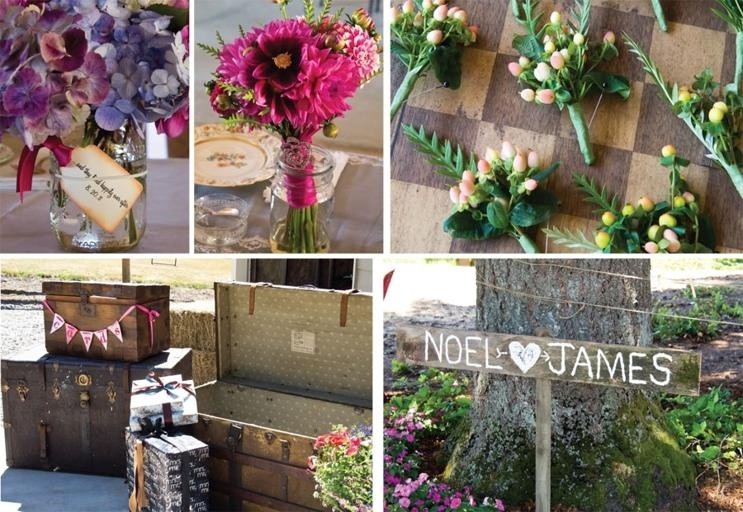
[193,191,250,248]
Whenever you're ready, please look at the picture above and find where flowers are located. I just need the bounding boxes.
[389,0,743,254]
[194,1,383,252]
[0,0,189,243]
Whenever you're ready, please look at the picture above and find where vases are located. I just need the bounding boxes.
[48,121,149,252]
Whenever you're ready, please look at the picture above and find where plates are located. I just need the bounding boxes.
[194,121,285,189]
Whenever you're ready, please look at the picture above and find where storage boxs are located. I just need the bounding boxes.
[1,346,193,477]
[195,281,373,512]
[41,280,170,364]
